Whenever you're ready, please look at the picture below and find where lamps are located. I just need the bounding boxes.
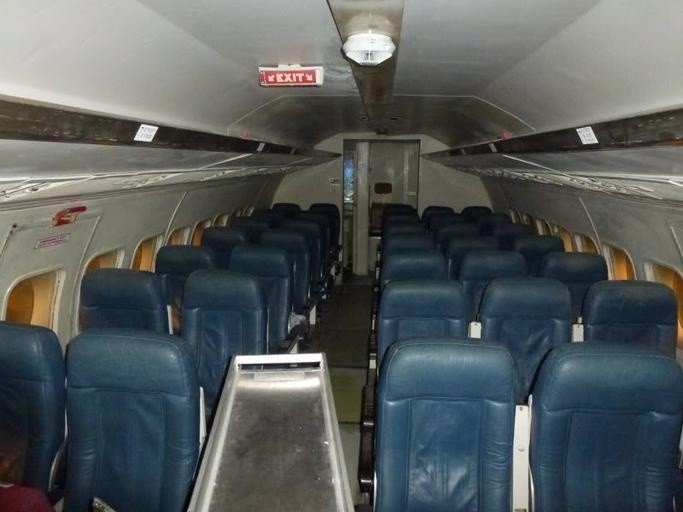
[342,34,395,65]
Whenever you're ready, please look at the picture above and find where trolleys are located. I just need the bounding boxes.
[183,352,356,508]
